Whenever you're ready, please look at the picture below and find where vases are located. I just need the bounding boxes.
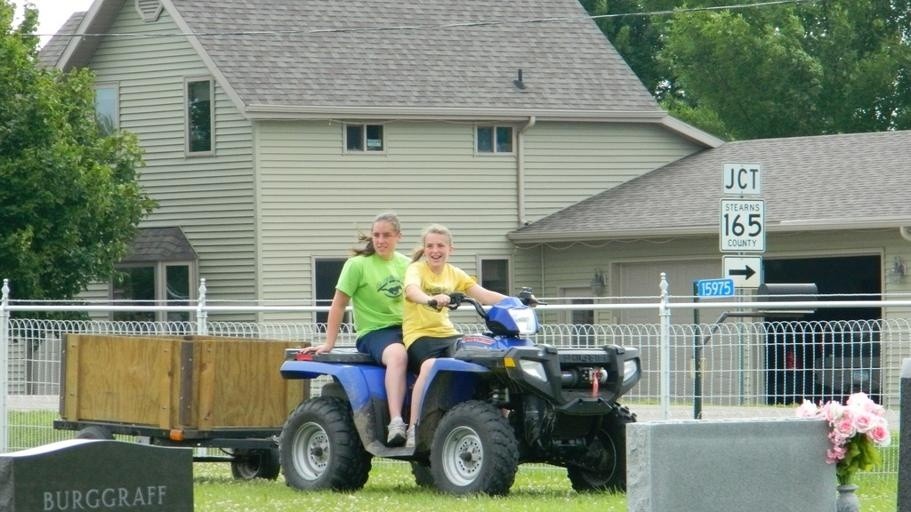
[837,485,859,512]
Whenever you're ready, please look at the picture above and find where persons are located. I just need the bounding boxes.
[402,223,541,448]
[297,213,420,444]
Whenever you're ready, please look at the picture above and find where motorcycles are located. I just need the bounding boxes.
[280,287,641,499]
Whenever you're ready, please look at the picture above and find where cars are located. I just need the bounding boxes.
[786,327,879,404]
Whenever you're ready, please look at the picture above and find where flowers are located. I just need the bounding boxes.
[795,392,892,486]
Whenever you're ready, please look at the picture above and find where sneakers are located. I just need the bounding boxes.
[405,422,418,449]
[388,419,407,447]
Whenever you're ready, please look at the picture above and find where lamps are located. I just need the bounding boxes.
[591,267,604,297]
[888,256,905,284]
